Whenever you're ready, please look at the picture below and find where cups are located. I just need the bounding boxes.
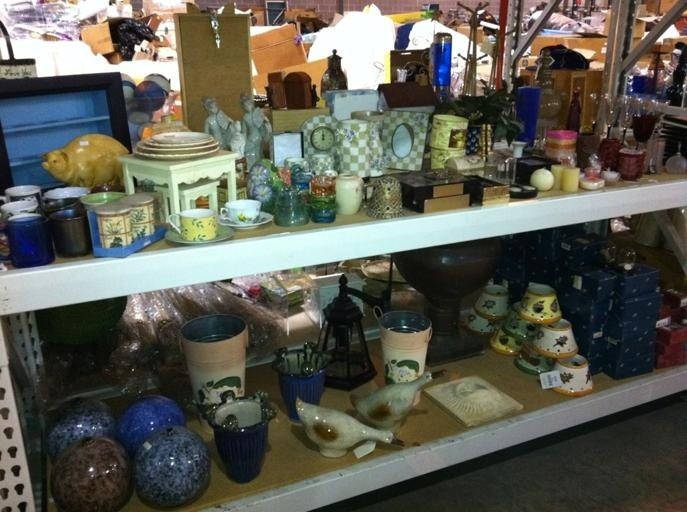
[219,199,262,226]
[308,176,337,223]
[40,186,93,217]
[48,210,94,257]
[179,313,250,422]
[1,214,56,268]
[1,200,41,221]
[377,311,433,408]
[619,145,648,182]
[599,95,618,136]
[632,96,656,152]
[273,191,310,228]
[576,132,599,180]
[613,94,637,147]
[4,184,42,201]
[82,191,129,210]
[167,208,218,241]
[601,137,621,174]
[589,91,608,136]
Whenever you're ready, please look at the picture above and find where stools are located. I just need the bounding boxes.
[156,179,219,222]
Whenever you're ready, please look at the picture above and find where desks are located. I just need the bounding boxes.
[117,148,238,230]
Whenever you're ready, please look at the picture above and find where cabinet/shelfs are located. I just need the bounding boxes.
[0,0,687,512]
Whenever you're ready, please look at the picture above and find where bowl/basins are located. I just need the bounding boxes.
[489,325,525,356]
[502,311,541,341]
[473,284,510,322]
[516,283,562,326]
[550,352,594,398]
[530,316,579,360]
[513,340,554,377]
[462,311,498,337]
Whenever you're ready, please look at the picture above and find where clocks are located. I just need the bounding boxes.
[302,115,346,175]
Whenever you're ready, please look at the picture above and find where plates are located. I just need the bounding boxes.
[217,211,275,230]
[136,141,217,154]
[166,226,236,246]
[133,147,218,160]
[153,130,213,147]
